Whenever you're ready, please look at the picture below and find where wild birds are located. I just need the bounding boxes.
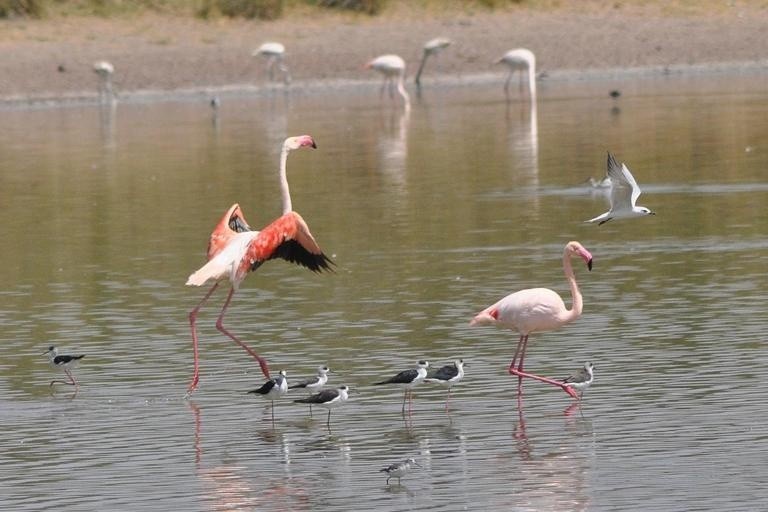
[249,365,352,425]
[495,47,537,98]
[582,149,657,226]
[414,38,452,85]
[558,360,596,400]
[92,62,115,97]
[363,53,410,103]
[252,42,292,84]
[184,134,340,391]
[40,345,87,388]
[370,358,469,410]
[469,240,598,401]
